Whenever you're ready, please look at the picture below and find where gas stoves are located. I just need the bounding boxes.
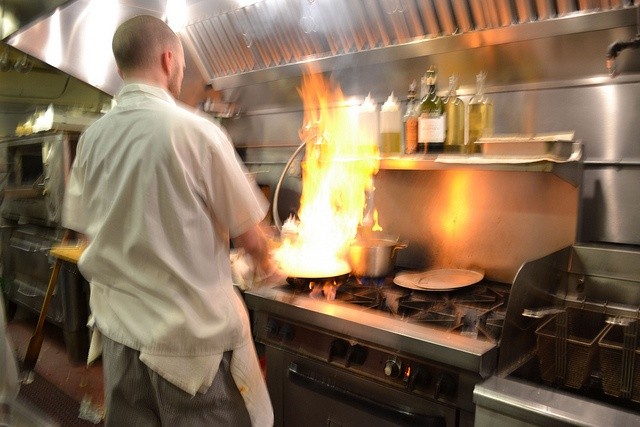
[274,263,512,344]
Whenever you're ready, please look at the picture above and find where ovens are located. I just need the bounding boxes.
[281,352,460,427]
[0,122,82,333]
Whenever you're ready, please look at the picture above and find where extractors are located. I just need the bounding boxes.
[181,0,629,93]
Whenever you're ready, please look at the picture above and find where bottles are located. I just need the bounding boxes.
[381,93,400,155]
[469,73,493,152]
[403,85,417,152]
[364,91,377,155]
[445,74,464,154]
[417,66,445,151]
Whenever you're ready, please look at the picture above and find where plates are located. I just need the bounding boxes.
[413,270,484,289]
[393,273,451,292]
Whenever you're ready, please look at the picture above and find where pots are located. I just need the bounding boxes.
[268,264,352,291]
[350,235,410,277]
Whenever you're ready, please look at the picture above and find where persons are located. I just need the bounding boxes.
[60,15,273,426]
